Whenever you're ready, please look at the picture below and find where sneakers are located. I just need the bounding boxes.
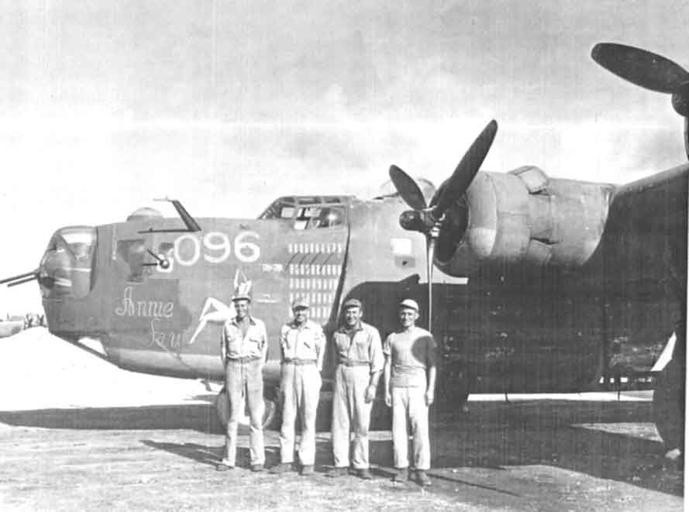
[216,464,373,480]
[394,470,432,485]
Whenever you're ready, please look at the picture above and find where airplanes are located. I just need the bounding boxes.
[0,42,688,447]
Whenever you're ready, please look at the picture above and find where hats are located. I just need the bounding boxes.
[399,299,419,311]
[232,295,251,303]
[343,299,362,310]
[292,297,309,310]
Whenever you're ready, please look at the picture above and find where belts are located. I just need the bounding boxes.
[295,360,315,365]
[229,357,257,363]
[339,362,369,367]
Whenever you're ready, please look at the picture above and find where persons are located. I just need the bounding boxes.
[325,297,386,481]
[269,295,328,477]
[215,291,270,471]
[379,298,437,486]
